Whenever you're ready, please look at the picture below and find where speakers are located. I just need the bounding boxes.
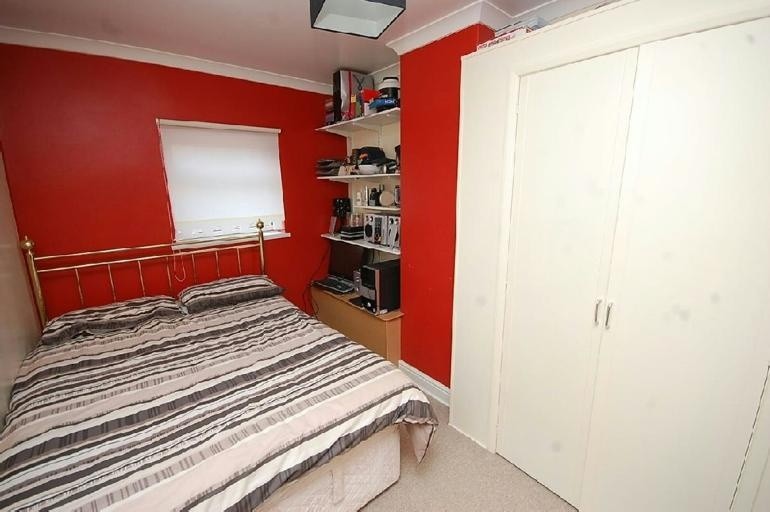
[362,213,374,243]
[388,214,401,249]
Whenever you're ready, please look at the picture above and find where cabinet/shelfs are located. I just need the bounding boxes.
[314,105,408,256]
[445,0,770,512]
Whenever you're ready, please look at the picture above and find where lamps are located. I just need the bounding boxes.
[306,0,409,38]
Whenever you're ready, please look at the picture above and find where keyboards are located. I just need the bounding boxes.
[314,278,354,294]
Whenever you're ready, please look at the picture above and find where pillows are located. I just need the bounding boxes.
[39,275,287,348]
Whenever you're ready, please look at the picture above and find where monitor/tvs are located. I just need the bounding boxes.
[328,240,368,282]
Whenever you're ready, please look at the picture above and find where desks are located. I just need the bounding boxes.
[308,276,402,361]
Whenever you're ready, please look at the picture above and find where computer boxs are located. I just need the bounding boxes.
[359,259,400,315]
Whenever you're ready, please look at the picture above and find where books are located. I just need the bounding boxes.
[340,233,364,241]
[339,225,364,235]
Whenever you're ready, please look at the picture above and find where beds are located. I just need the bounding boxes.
[0,214,414,512]
